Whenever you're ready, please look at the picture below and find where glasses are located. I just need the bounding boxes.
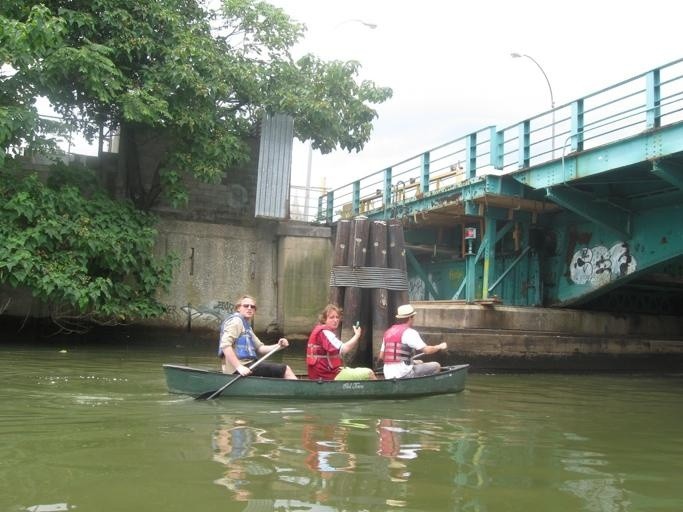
[244,304,256,308]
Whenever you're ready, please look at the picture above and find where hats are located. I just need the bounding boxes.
[395,304,417,319]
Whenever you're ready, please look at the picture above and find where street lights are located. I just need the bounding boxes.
[509,50,555,160]
[301,16,377,224]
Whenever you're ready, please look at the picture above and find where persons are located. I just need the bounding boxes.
[379,304,447,380]
[305,303,377,381]
[216,295,296,380]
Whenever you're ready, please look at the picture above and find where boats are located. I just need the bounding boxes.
[162,362,470,399]
[169,389,459,437]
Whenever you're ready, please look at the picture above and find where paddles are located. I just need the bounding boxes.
[195,347,280,401]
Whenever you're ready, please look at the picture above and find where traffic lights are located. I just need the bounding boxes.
[462,226,476,240]
